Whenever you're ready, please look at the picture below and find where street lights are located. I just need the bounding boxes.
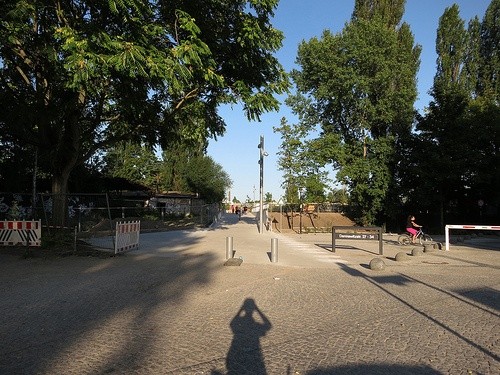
[258,134,264,235]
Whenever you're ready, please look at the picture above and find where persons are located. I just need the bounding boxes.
[231,204,248,215]
[406,215,422,244]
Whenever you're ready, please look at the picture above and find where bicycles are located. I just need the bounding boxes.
[398,227,432,247]
